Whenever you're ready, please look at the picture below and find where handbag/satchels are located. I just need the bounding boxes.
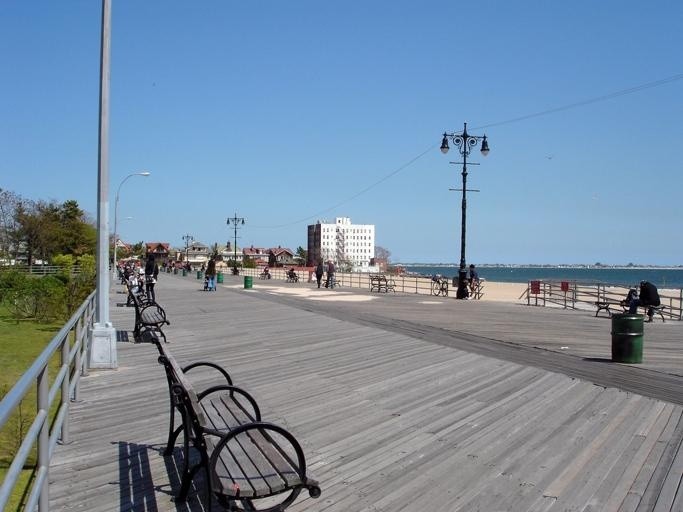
[145,274,154,282]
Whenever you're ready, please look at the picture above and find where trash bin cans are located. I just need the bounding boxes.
[182,268,187,276]
[611,314,644,363]
[174,268,178,275]
[244,276,251,288]
[196,271,201,279]
[217,272,223,283]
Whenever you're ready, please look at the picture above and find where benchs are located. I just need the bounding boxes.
[240,422,246,424]
[593,300,665,324]
[369,274,397,293]
[118,268,170,343]
[154,335,321,512]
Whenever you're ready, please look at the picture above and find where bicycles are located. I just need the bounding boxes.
[432,272,449,297]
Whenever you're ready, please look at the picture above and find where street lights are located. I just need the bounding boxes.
[440,121,490,300]
[226,212,244,275]
[182,233,194,264]
[112,171,151,280]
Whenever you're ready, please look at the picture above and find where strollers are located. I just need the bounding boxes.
[203,271,217,292]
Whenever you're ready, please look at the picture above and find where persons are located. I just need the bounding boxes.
[288,268,297,278]
[234,264,238,275]
[316,262,323,288]
[626,285,638,300]
[325,260,335,289]
[131,255,216,302]
[467,264,479,299]
[264,267,270,279]
[627,280,660,322]
